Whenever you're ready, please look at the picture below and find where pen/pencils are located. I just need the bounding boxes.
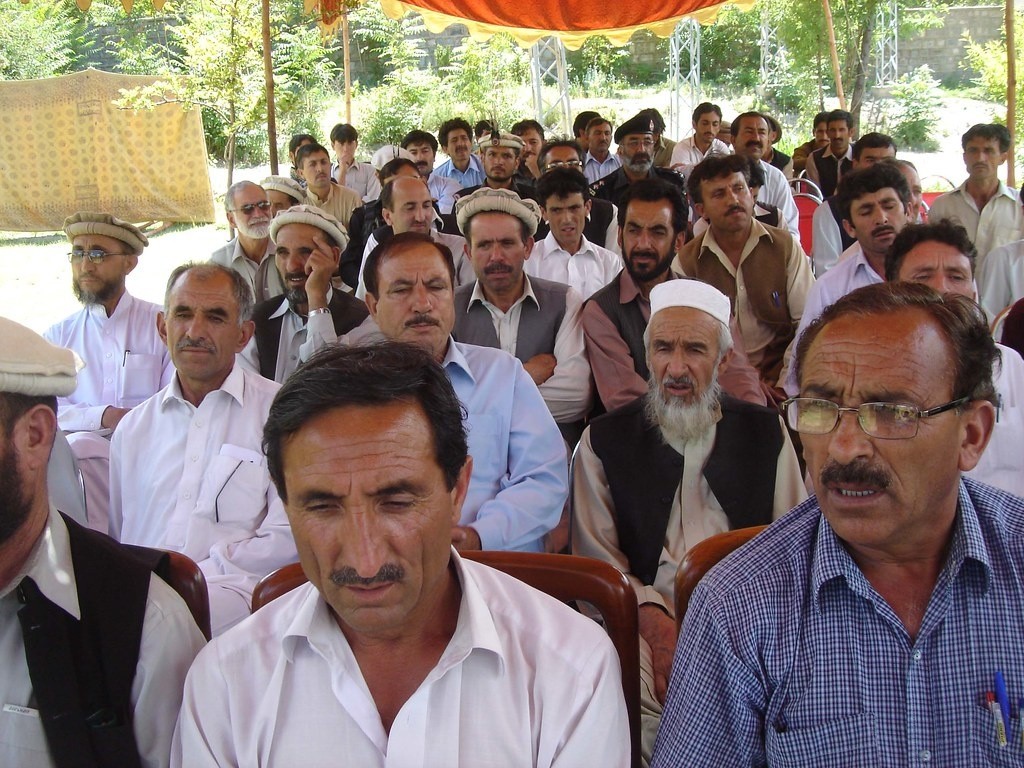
[123,350,131,367]
[772,290,779,306]
[993,702,1007,746]
[986,691,995,712]
[997,394,1002,423]
[994,672,1011,743]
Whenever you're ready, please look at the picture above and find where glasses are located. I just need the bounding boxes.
[628,140,656,149]
[230,201,272,215]
[544,160,582,171]
[779,395,972,441]
[67,249,131,264]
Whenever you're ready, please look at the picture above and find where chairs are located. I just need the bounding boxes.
[788,168,955,259]
[159,527,770,768]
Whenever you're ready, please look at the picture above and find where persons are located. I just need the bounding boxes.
[567,277,810,767]
[0,314,212,768]
[883,216,1024,500]
[168,336,628,766]
[362,231,570,555]
[448,187,593,453]
[668,155,817,417]
[108,261,301,643]
[784,163,910,400]
[210,106,1024,309]
[980,238,1024,340]
[250,204,368,385]
[647,281,1024,768]
[583,179,771,412]
[39,210,176,441]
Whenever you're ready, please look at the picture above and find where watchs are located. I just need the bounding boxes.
[309,307,331,317]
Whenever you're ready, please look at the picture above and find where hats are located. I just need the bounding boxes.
[455,186,542,236]
[372,145,417,171]
[269,203,351,253]
[0,317,78,398]
[478,128,526,153]
[260,175,307,206]
[64,211,149,256]
[649,278,732,330]
[614,114,655,144]
